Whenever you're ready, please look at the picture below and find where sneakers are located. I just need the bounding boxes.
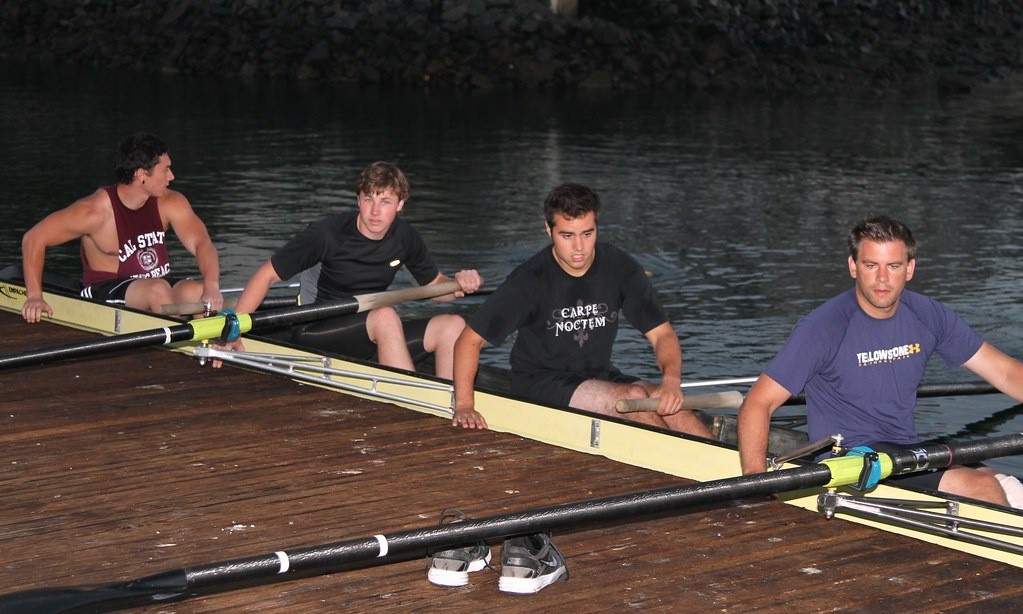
[496,530,570,595]
[427,539,491,588]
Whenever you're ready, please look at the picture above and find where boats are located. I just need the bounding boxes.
[0,264,1023,614]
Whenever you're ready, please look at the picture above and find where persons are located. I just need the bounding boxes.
[21,133,226,325]
[447,180,716,443]
[733,213,1023,515]
[203,160,486,381]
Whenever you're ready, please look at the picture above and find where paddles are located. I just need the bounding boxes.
[616,377,1005,411]
[154,293,298,314]
[0,279,462,370]
[0,431,1023,614]
